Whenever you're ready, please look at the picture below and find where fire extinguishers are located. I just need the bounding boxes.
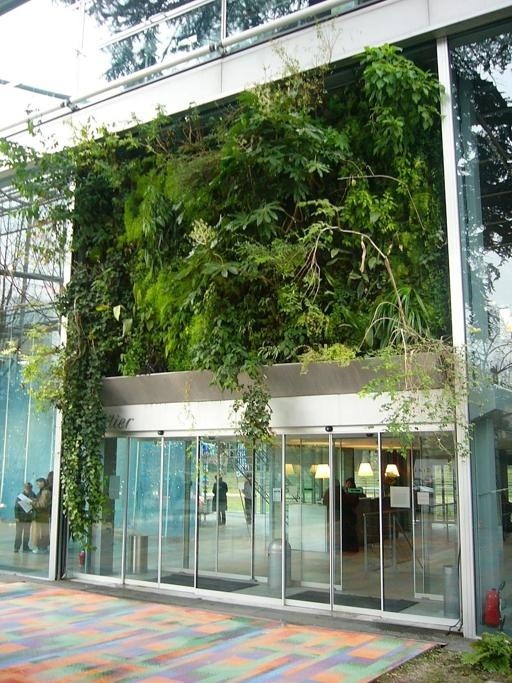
[79,552,86,566]
[483,581,505,627]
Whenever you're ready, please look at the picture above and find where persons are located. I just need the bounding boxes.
[241,472,256,524]
[211,474,229,526]
[13,482,37,553]
[323,477,348,521]
[46,470,54,492]
[28,478,50,554]
[342,477,360,553]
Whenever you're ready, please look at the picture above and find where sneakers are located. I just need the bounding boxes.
[14,548,48,554]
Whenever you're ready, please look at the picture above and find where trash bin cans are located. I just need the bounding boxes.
[443,565,461,619]
[268,540,291,587]
[131,535,149,574]
[92,521,112,573]
